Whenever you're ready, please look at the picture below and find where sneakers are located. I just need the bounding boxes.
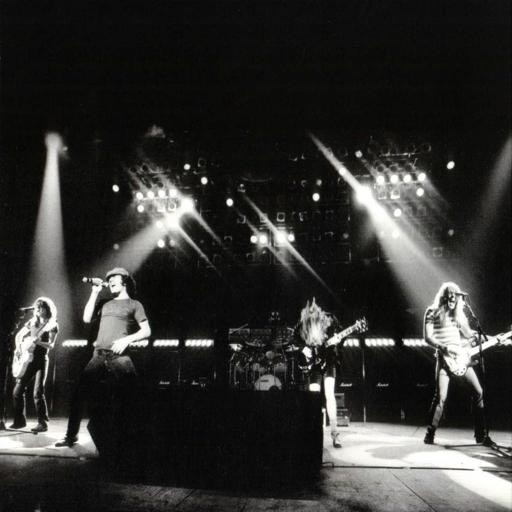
[31,423,49,432]
[55,439,73,447]
[9,423,26,428]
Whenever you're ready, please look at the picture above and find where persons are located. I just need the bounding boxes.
[422,280,511,448]
[55,266,153,448]
[292,294,368,449]
[9,296,60,432]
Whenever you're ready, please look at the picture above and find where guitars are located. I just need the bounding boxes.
[297,317,368,376]
[11,322,57,378]
[438,332,512,378]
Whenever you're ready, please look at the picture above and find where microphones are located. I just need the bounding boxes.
[20,306,35,311]
[88,278,108,287]
[455,291,469,296]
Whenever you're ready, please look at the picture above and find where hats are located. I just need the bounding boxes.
[105,267,128,280]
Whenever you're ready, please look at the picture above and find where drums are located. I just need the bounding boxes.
[254,374,281,391]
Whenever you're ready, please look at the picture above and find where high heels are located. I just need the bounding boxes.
[331,432,342,448]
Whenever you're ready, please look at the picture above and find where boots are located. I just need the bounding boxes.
[424,424,436,444]
[475,429,486,443]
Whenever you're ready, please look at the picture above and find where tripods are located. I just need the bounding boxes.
[444,297,512,460]
[0,313,37,434]
